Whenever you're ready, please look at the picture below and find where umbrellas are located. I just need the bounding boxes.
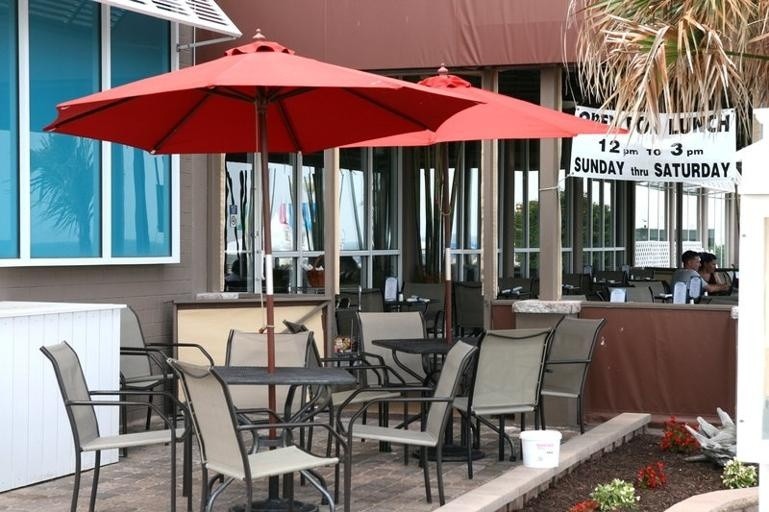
[338,62,630,350]
[44,28,486,451]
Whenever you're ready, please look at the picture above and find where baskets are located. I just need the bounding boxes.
[307,255,344,288]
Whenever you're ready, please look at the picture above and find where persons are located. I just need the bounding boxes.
[672,250,728,293]
[698,251,723,285]
[225,259,245,283]
[340,258,360,285]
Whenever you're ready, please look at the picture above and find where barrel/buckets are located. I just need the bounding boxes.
[518,431,562,469]
